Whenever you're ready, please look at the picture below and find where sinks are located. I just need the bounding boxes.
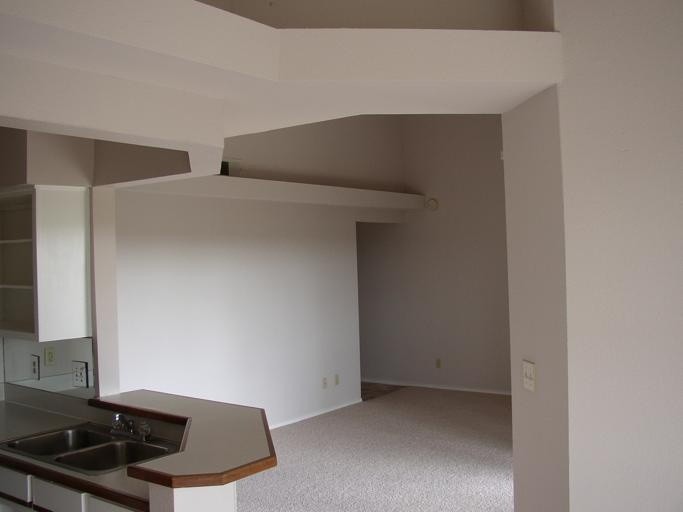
[0,429,114,454]
[54,440,179,478]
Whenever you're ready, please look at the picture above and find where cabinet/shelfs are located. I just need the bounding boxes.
[0,461,141,512]
[0,181,95,343]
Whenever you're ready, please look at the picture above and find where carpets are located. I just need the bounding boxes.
[357,379,406,402]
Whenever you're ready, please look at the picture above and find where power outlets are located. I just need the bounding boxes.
[28,353,40,382]
[69,361,88,389]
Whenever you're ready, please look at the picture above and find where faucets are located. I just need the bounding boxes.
[114,413,134,435]
